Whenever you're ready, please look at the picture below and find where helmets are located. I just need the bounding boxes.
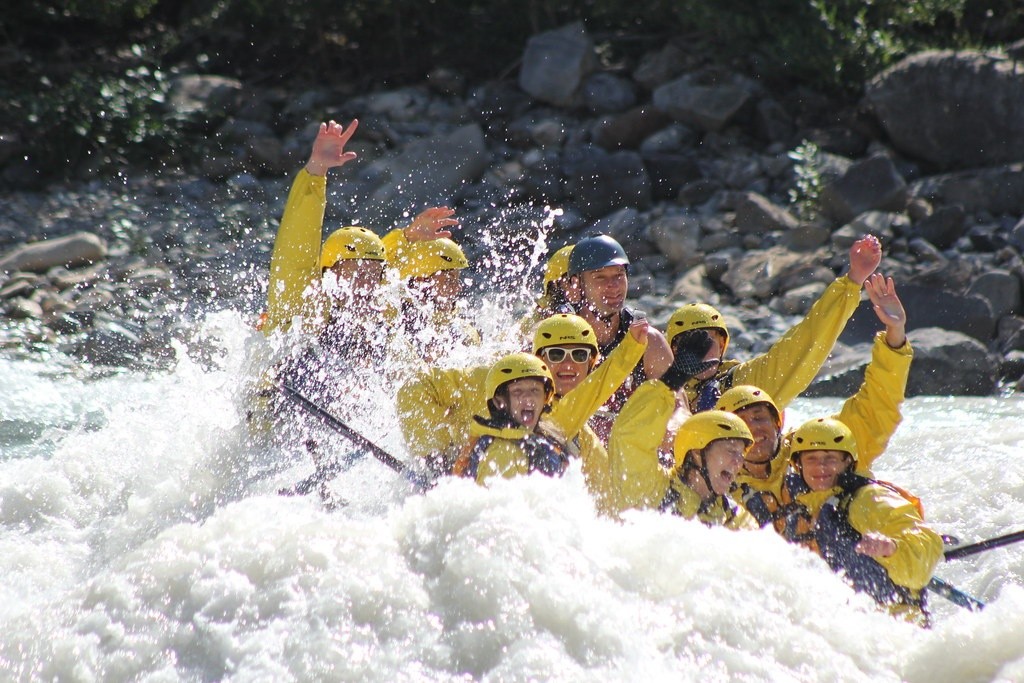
[321,227,385,277]
[485,353,556,428]
[666,304,730,358]
[673,409,755,472]
[531,313,598,371]
[788,418,859,473]
[715,384,783,432]
[567,232,630,283]
[542,245,577,298]
[400,237,469,279]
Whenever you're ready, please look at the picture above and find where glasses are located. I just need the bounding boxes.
[540,347,591,364]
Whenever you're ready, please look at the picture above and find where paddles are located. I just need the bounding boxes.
[923,575,989,615]
[943,529,1024,565]
[264,372,434,511]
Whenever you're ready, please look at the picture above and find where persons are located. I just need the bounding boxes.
[241,116,943,633]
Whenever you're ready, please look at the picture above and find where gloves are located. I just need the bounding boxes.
[659,329,720,394]
